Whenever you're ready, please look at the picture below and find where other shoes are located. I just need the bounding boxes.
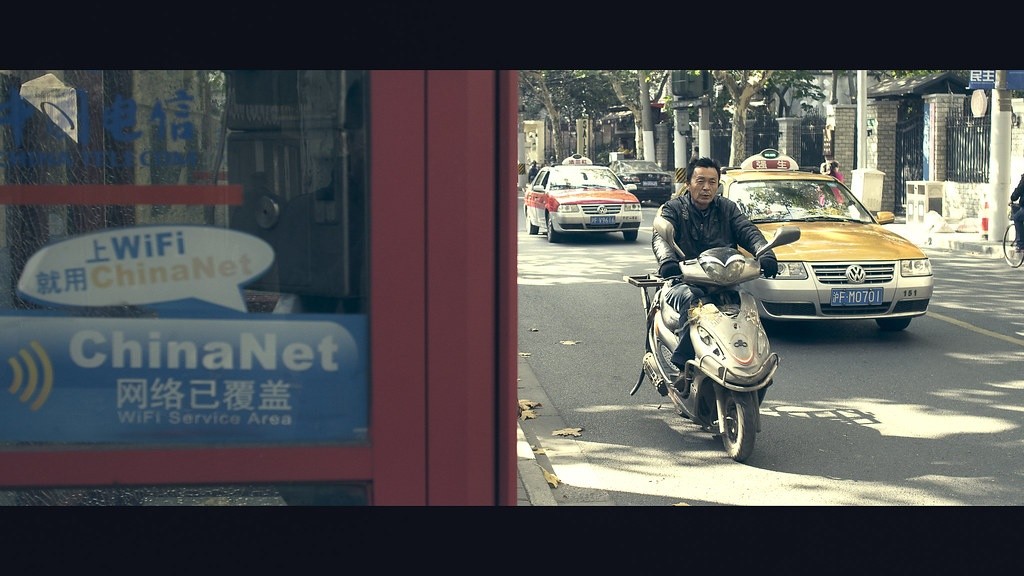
[1015,245,1024,252]
[674,372,685,391]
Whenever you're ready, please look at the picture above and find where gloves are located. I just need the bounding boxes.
[660,261,683,280]
[760,257,778,279]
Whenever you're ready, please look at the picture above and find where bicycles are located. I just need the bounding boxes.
[1003,203,1024,268]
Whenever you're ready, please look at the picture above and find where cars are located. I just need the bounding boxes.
[524,153,642,243]
[672,148,932,330]
[605,160,675,205]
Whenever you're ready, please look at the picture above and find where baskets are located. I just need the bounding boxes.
[1010,203,1020,218]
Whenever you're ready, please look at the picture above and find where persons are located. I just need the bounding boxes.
[264,76,368,314]
[825,162,845,204]
[651,158,778,394]
[1011,174,1023,253]
[688,146,698,162]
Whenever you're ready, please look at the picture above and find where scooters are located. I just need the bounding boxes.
[624,216,801,462]
